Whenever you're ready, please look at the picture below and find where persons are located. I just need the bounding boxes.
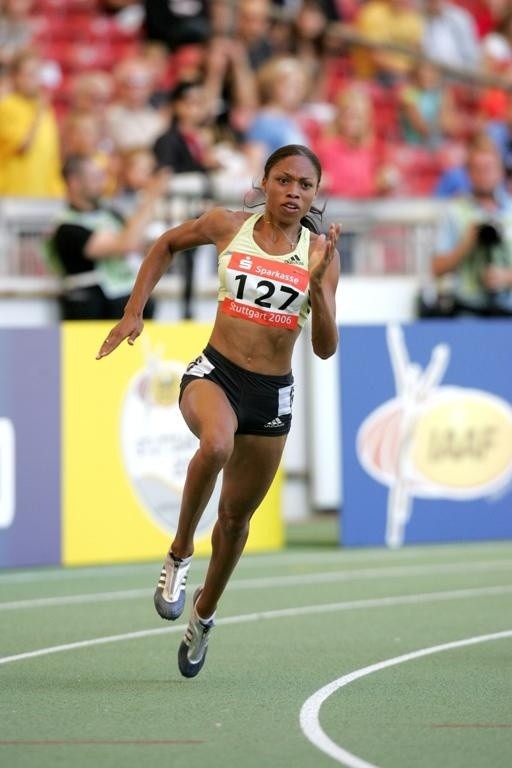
[420,131,512,320]
[96,145,339,681]
[51,145,166,318]
[2,0,511,283]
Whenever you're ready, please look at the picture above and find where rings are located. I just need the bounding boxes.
[104,338,110,343]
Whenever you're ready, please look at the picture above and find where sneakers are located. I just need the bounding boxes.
[150,544,196,621]
[174,581,216,680]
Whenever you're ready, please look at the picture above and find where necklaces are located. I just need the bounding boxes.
[266,215,303,249]
